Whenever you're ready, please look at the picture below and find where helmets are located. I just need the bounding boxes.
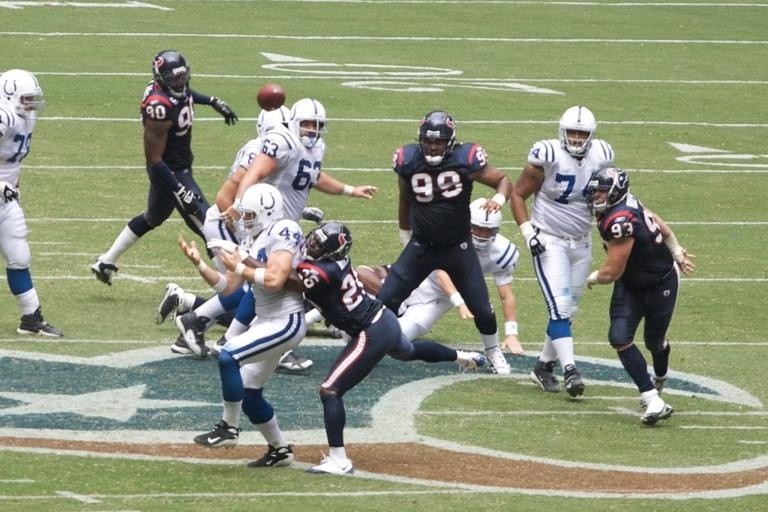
[583,167,629,217]
[560,105,595,158]
[417,110,456,166]
[235,98,353,262]
[0,69,44,121]
[469,197,503,250]
[152,50,189,100]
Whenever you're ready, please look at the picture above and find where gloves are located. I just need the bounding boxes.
[1,181,19,203]
[207,237,247,261]
[212,97,236,124]
[519,222,546,256]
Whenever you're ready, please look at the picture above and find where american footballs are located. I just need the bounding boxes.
[257,84,286,111]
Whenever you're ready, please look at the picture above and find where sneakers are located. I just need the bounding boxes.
[462,346,674,424]
[173,181,204,216]
[156,283,354,474]
[17,307,62,338]
[90,259,118,284]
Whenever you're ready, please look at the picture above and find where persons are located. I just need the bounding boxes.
[90,48,240,287]
[509,106,616,396]
[176,183,487,473]
[0,67,65,340]
[373,109,523,375]
[157,97,379,378]
[585,167,697,425]
